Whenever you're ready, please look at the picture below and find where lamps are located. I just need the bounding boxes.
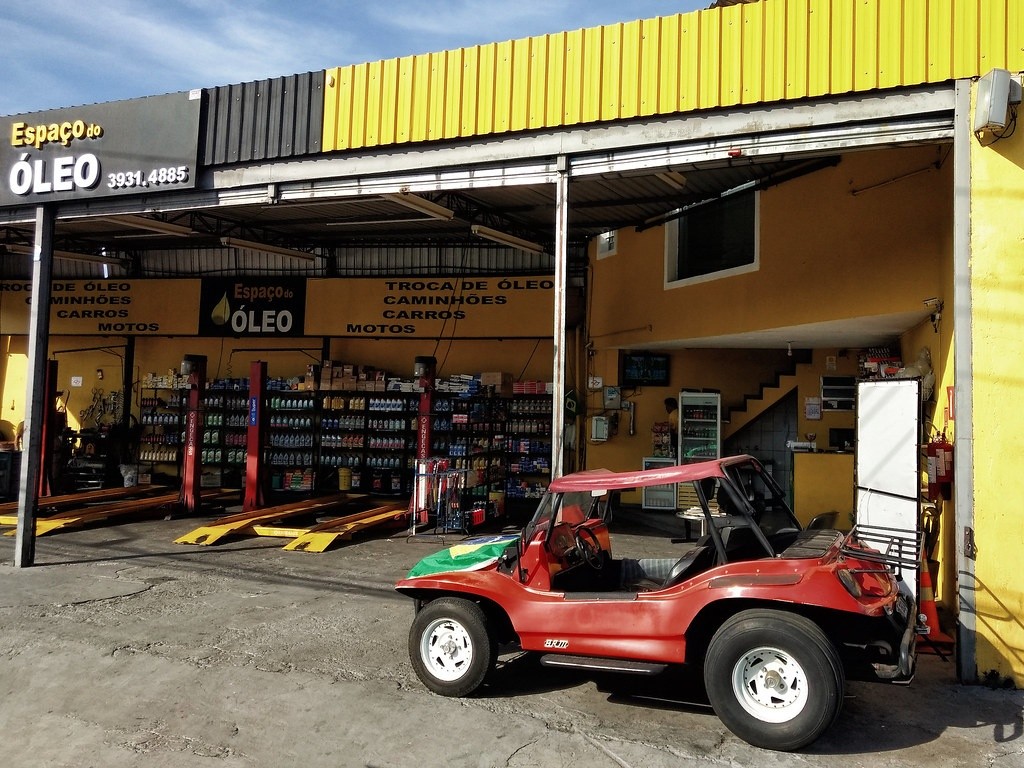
[216,228,318,264]
[787,342,793,357]
[95,215,193,239]
[655,170,688,190]
[1,232,123,268]
[378,193,453,222]
[472,224,544,256]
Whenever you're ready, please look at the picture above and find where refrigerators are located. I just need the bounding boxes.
[677,388,721,512]
[641,455,677,510]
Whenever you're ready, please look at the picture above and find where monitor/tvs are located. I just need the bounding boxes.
[829,428,856,453]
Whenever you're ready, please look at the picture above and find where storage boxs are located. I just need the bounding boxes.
[322,367,333,379]
[359,374,369,381]
[357,382,366,390]
[495,385,511,394]
[375,381,388,391]
[332,367,343,377]
[366,381,375,391]
[331,378,344,390]
[304,377,319,389]
[468,381,481,395]
[320,379,332,389]
[344,376,357,390]
[324,360,335,367]
[343,369,355,376]
[460,374,474,383]
[449,374,462,386]
[138,472,165,484]
[400,383,414,392]
[306,364,321,378]
[298,383,304,390]
[481,372,513,385]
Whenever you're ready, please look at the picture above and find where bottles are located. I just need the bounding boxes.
[457,400,511,432]
[143,432,179,443]
[183,397,187,405]
[205,395,249,410]
[181,428,186,442]
[270,415,419,430]
[263,450,415,469]
[512,399,551,413]
[432,397,453,450]
[269,433,418,449]
[512,418,551,433]
[141,394,180,406]
[183,414,186,425]
[142,413,178,424]
[450,435,530,529]
[140,449,177,461]
[271,395,419,411]
[205,412,249,426]
[202,447,247,463]
[203,428,247,445]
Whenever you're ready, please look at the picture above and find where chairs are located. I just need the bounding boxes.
[626,530,721,593]
[661,543,713,591]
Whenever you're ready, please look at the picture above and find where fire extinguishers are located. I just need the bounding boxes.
[937,425,954,482]
[924,420,943,503]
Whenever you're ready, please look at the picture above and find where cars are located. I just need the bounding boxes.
[392,451,932,753]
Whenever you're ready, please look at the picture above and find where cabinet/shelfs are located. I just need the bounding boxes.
[136,388,183,486]
[404,392,457,499]
[180,388,232,489]
[221,390,269,488]
[366,391,409,498]
[317,390,366,494]
[508,394,554,504]
[458,395,507,504]
[268,390,318,493]
